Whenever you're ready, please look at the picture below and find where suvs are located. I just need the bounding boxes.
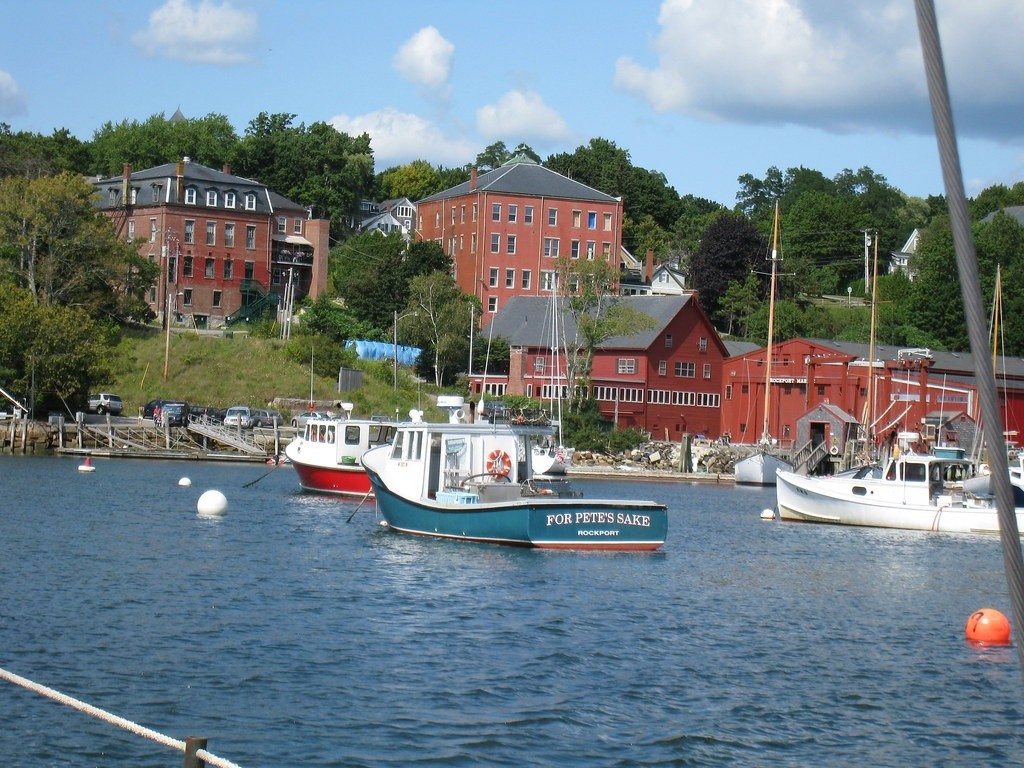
[87,394,123,415]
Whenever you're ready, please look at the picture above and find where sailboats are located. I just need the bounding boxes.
[528,278,575,475]
[808,230,1023,496]
[733,199,793,487]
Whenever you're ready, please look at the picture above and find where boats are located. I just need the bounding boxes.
[284,415,401,498]
[362,394,669,550]
[776,454,1023,536]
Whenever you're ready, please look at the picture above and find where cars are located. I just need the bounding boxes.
[481,401,510,419]
[291,411,333,428]
[142,399,283,429]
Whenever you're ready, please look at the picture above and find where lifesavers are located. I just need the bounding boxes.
[540,489,553,495]
[830,446,839,455]
[1008,449,1015,458]
[324,431,333,443]
[518,416,523,422]
[487,450,511,479]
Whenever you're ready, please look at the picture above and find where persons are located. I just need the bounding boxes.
[154,405,161,426]
[137,404,144,426]
[724,428,732,444]
